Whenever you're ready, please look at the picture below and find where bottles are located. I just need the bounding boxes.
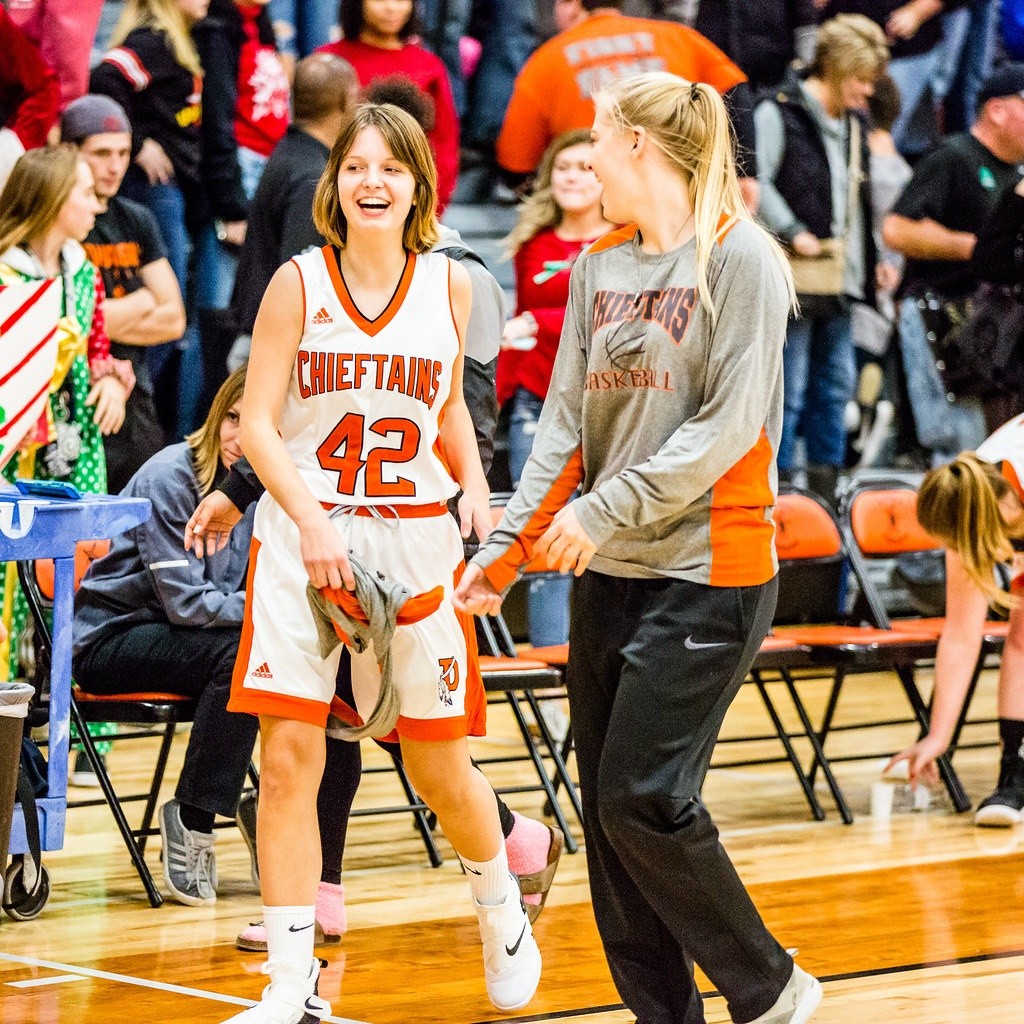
[883,777,949,813]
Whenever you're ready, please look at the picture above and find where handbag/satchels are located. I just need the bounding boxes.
[779,238,843,295]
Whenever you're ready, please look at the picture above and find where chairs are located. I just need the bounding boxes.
[18,479,1013,909]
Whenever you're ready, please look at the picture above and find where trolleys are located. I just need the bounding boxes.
[0,479,153,920]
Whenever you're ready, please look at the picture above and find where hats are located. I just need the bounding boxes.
[60,94,133,142]
[977,64,1024,100]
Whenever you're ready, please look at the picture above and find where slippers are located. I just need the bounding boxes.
[236,918,341,951]
[517,826,564,924]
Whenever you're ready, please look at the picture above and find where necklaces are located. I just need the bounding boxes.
[633,212,694,308]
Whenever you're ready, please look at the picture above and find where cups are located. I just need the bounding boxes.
[871,782,894,817]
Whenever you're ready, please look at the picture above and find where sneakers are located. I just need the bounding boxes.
[745,948,823,1024]
[235,789,260,889]
[71,751,111,786]
[472,871,542,1011]
[974,757,1024,826]
[159,798,219,907]
[523,701,575,748]
[217,957,332,1024]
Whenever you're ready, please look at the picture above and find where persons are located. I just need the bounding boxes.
[0,0,1024,1023]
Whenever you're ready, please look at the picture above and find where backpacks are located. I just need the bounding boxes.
[911,136,1024,402]
